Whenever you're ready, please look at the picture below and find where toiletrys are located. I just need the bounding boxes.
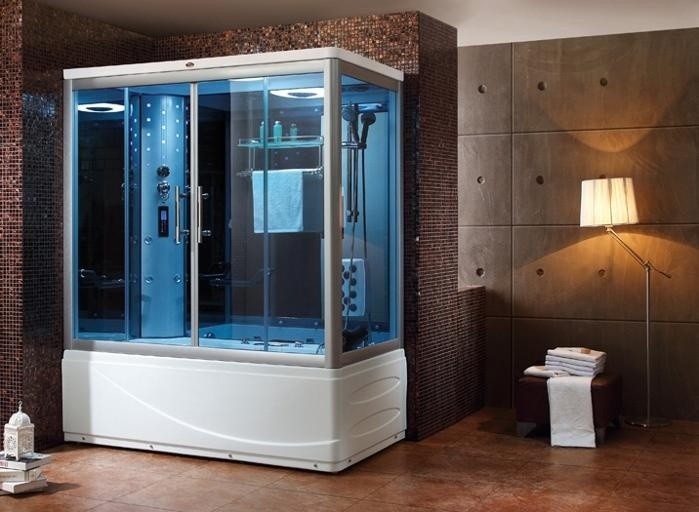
[273,120,283,144]
[259,121,266,144]
[290,123,297,141]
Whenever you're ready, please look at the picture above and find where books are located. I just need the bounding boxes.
[0,449,52,471]
[0,474,49,494]
[0,483,49,496]
[0,466,43,483]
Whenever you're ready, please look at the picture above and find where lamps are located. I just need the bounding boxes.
[579,174,673,430]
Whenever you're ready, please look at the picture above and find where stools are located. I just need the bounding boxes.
[514,375,622,431]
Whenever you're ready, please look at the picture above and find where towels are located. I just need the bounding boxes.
[251,167,305,234]
[522,345,606,449]
